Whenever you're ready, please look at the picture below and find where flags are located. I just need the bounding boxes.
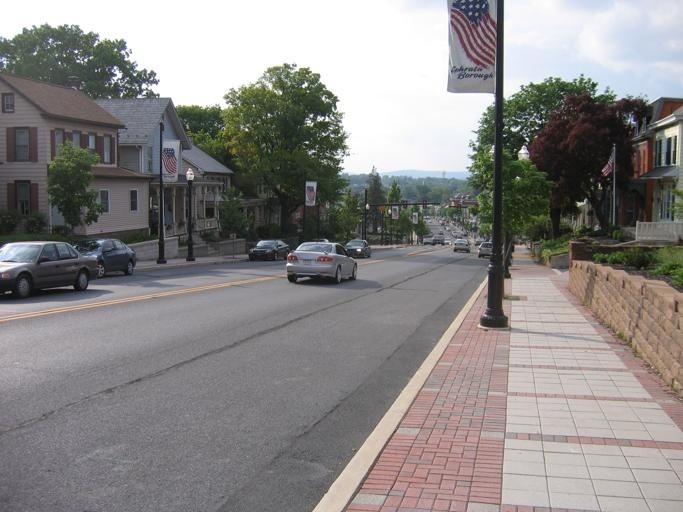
[601,147,614,177]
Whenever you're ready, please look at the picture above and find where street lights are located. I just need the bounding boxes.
[361,203,371,240]
[186,167,195,261]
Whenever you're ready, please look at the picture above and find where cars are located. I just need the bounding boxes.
[478,242,493,258]
[0,238,136,298]
[454,239,471,253]
[432,234,445,246]
[286,241,357,283]
[248,238,372,261]
